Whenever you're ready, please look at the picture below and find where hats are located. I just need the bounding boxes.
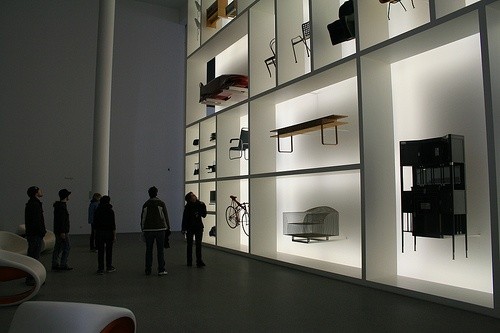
[27,186,40,197]
[59,189,71,201]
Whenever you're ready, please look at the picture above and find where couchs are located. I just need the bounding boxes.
[328,0,355,45]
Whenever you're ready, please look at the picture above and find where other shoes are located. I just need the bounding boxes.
[145,269,151,275]
[186,264,192,266]
[197,263,205,268]
[107,267,116,272]
[97,270,104,275]
[159,272,169,275]
[90,248,98,252]
[51,265,72,270]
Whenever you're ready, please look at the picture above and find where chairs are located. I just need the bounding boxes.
[264,38,276,77]
[0,230,30,282]
[291,21,311,62]
[16,225,56,253]
[228,127,249,160]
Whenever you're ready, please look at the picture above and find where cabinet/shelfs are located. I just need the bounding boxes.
[398,135,467,259]
[207,0,228,28]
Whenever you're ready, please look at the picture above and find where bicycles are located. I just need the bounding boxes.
[225,195,250,237]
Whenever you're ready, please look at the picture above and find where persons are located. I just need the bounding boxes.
[93,195,119,274]
[141,185,171,274]
[52,188,73,272]
[87,193,102,252]
[24,185,47,279]
[181,191,207,267]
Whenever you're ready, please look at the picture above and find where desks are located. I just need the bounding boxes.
[270,115,349,153]
